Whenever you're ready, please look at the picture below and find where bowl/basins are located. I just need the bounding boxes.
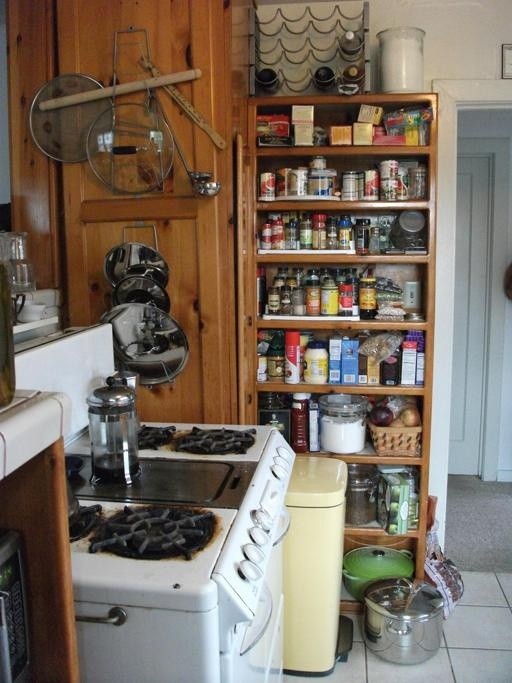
[0,230,46,323]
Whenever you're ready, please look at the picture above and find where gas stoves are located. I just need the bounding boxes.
[65,421,296,655]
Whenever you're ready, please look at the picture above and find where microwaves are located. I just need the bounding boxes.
[0,527,33,683]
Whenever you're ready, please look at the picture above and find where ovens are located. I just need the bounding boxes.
[73,504,291,683]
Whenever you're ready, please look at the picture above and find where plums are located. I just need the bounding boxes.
[370,407,393,426]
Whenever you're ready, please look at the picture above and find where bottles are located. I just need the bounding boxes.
[257,263,377,320]
[346,464,380,526]
[257,329,328,385]
[376,26,426,94]
[316,66,336,92]
[257,156,428,204]
[259,68,278,94]
[261,211,391,255]
[341,30,364,62]
[259,392,370,454]
[342,65,365,87]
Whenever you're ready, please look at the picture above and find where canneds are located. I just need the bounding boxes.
[259,156,408,203]
[360,277,376,320]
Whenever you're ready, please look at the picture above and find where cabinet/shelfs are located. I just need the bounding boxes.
[249,2,371,95]
[1,389,79,683]
[246,94,438,612]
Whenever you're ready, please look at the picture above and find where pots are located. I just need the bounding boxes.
[364,576,444,667]
[342,543,416,603]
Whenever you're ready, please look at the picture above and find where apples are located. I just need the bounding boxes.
[389,396,422,428]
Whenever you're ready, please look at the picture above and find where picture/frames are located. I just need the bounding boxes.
[502,44,512,79]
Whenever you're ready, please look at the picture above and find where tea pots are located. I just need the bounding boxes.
[89,375,142,487]
